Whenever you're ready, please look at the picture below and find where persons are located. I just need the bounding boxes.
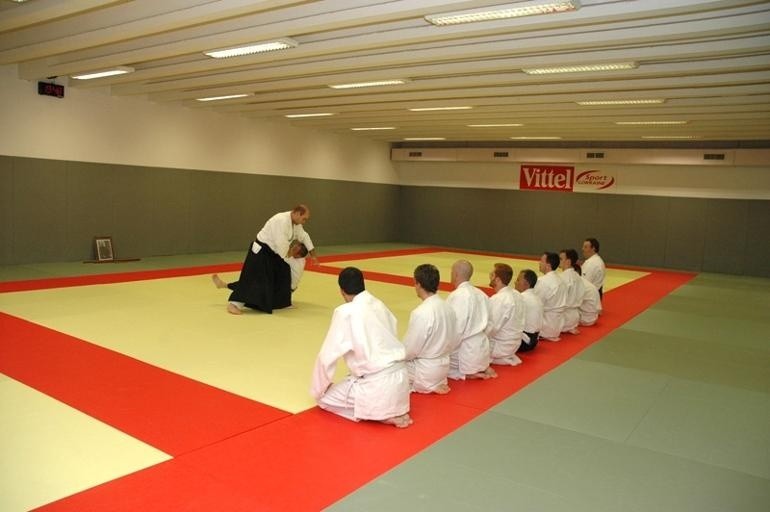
[580,238,606,301]
[514,269,544,352]
[402,264,456,395]
[533,251,568,342]
[227,204,320,315]
[310,266,414,429]
[574,264,603,326]
[557,249,583,334]
[488,264,525,366]
[212,243,308,296]
[445,259,498,380]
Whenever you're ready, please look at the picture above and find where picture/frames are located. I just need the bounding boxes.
[94,236,114,263]
